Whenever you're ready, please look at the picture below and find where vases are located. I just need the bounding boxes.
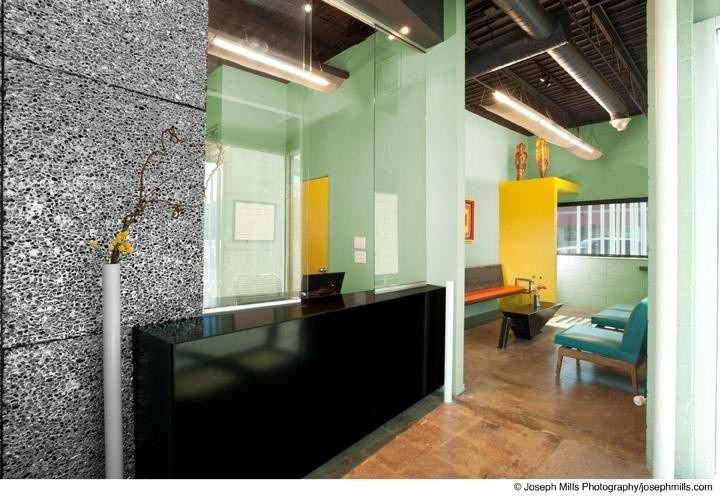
[100,261,125,479]
[534,294,542,307]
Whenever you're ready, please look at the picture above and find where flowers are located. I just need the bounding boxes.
[531,273,549,295]
[88,119,224,264]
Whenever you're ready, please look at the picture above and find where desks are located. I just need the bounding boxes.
[497,300,564,350]
[131,280,448,480]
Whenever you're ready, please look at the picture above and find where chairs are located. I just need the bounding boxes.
[553,296,647,396]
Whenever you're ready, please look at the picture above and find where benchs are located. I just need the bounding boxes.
[464,264,524,306]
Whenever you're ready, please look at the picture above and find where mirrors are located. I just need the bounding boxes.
[375,22,428,293]
[203,0,377,315]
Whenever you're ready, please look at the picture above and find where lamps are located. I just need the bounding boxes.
[205,25,350,95]
[475,70,607,162]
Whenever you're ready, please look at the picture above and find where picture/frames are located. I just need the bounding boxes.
[465,197,477,243]
[231,198,277,243]
